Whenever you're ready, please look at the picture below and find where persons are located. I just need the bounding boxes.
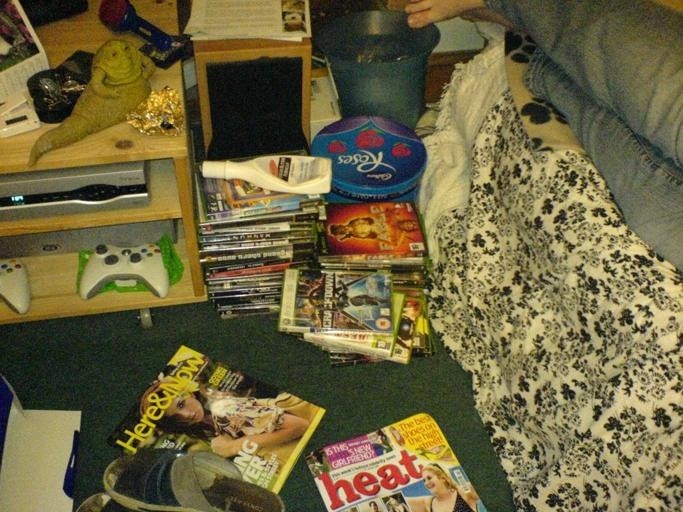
[384,0,683,274]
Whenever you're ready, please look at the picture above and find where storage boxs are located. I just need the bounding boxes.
[310,115,427,207]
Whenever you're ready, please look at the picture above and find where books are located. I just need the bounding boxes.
[193,150,438,365]
[105,344,326,494]
[304,412,487,512]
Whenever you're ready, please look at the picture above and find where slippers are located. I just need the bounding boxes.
[76,453,285,512]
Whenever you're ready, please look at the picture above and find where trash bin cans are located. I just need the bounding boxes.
[319,10,441,131]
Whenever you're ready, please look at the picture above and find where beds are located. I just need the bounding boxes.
[410,36,682,511]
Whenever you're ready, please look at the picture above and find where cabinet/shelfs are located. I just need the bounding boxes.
[0,0,208,329]
[191,32,313,161]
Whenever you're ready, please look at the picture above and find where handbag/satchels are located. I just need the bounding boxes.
[245,396,319,469]
